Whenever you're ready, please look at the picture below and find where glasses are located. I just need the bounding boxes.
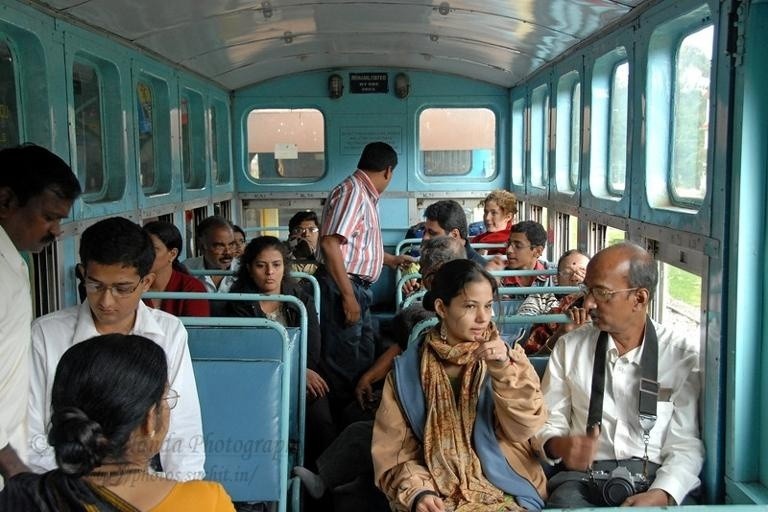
[559,269,641,303]
[292,227,319,234]
[162,389,180,411]
[83,277,143,297]
[504,241,540,250]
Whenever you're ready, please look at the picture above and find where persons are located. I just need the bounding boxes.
[1,331,236,511]
[527,239,708,509]
[1,140,83,496]
[123,187,592,511]
[18,215,209,491]
[304,139,399,446]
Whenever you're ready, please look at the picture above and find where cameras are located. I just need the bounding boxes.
[580,467,650,508]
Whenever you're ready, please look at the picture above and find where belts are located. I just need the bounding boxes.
[348,274,372,288]
